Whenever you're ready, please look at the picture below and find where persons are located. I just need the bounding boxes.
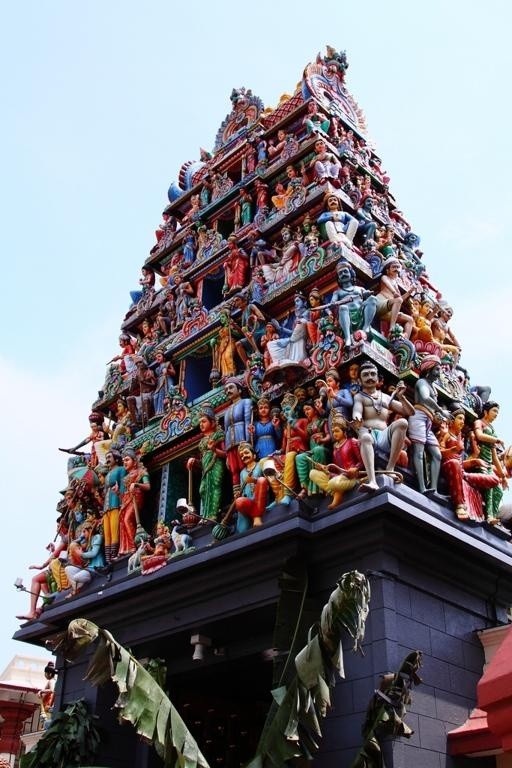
[14,97,510,624]
[36,660,58,731]
